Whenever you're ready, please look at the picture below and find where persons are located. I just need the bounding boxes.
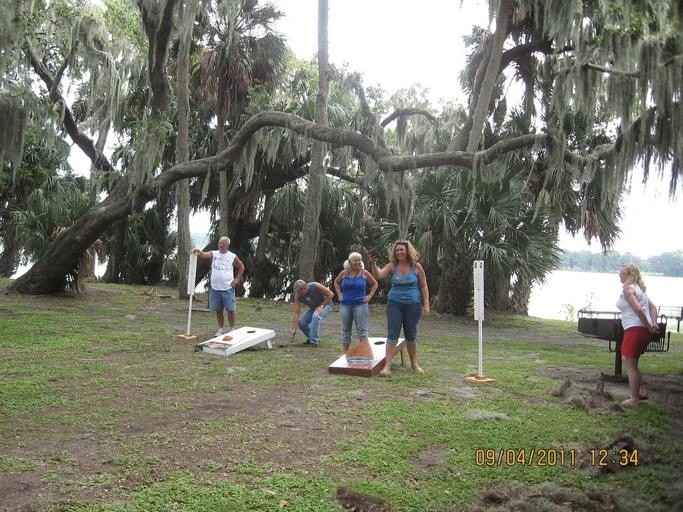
[614,264,661,406]
[190,236,245,338]
[334,252,379,354]
[369,240,430,376]
[290,278,334,348]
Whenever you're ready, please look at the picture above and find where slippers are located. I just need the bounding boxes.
[629,395,648,400]
[621,403,638,407]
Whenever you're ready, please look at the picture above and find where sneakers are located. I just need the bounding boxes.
[229,328,234,332]
[311,343,317,347]
[214,329,224,337]
[303,339,310,344]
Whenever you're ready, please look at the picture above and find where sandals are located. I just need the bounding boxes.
[379,369,390,376]
[413,368,424,373]
